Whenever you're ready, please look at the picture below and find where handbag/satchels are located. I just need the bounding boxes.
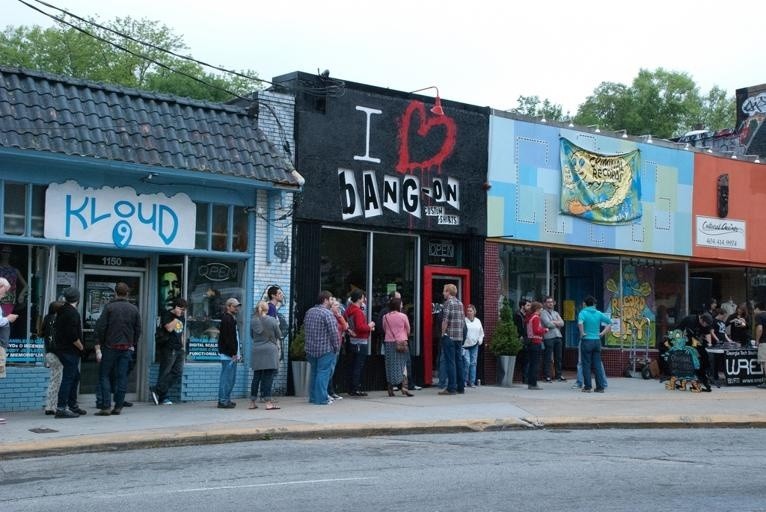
[397,339,408,352]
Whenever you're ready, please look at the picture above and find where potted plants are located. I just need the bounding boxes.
[290,321,311,398]
[491,296,525,384]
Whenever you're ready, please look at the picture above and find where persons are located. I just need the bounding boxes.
[216,296,242,408]
[676,296,765,388]
[93,282,141,416]
[438,285,485,394]
[95,353,134,408]
[39,301,63,415]
[249,284,423,411]
[0,244,27,314]
[159,270,180,311]
[0,275,19,425]
[148,299,187,407]
[51,288,88,420]
[203,285,225,319]
[514,295,613,393]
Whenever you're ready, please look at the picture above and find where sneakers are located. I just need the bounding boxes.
[54,407,79,419]
[414,385,422,390]
[121,400,134,408]
[528,375,606,393]
[150,386,159,405]
[96,402,107,409]
[95,408,112,416]
[0,417,9,424]
[393,386,400,391]
[438,381,477,395]
[309,390,369,405]
[217,400,237,408]
[43,409,56,415]
[69,405,87,415]
[162,399,172,405]
[110,408,121,415]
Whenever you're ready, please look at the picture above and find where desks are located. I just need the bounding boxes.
[706,348,758,385]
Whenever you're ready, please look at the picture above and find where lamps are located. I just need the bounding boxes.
[534,114,761,164]
[410,86,444,116]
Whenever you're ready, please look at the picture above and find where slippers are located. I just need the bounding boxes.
[248,396,281,409]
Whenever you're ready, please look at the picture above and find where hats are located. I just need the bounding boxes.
[226,297,242,307]
[63,287,80,303]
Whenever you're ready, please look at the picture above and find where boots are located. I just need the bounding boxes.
[401,375,415,398]
[387,382,395,397]
